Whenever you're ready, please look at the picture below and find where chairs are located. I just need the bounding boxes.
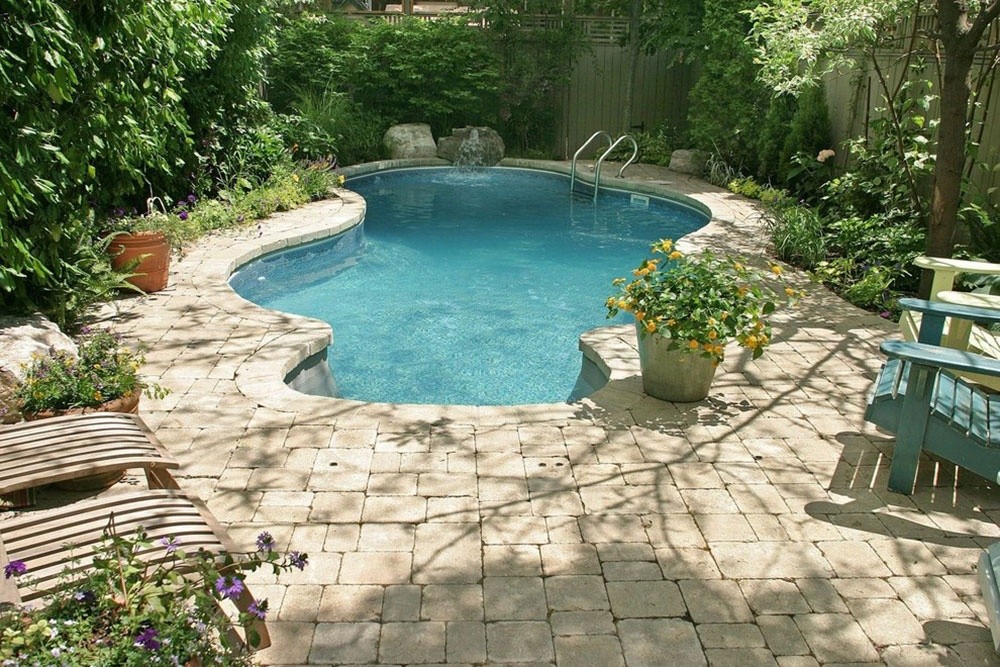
[864,258,1000,497]
[0,411,271,652]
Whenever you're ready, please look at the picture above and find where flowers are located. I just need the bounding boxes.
[606,235,805,364]
[12,331,174,403]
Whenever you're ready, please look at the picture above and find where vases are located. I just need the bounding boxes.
[20,385,141,495]
[634,309,723,404]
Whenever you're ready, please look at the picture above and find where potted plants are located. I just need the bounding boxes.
[105,213,175,294]
